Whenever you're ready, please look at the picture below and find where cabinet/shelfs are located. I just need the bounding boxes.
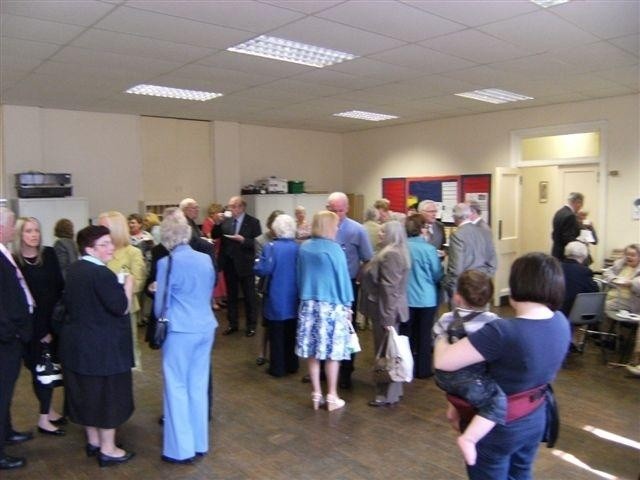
[11,198,92,248]
[239,194,328,236]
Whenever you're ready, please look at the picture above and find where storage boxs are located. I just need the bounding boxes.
[289,180,304,193]
[267,178,288,193]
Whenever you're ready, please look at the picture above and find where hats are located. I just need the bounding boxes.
[77,225,110,248]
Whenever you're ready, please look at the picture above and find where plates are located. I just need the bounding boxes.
[224,234,236,238]
[616,314,631,318]
[612,279,626,284]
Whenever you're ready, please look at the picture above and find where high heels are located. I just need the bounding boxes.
[311,392,345,411]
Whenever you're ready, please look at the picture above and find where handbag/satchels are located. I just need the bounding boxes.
[375,326,414,382]
[257,272,271,295]
[144,253,173,350]
[34,346,65,390]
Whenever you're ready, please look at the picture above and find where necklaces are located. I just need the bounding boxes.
[22,253,41,264]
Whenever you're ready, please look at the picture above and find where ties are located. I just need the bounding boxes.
[232,219,238,233]
[5,249,37,308]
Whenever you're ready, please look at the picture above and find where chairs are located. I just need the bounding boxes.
[568,294,604,355]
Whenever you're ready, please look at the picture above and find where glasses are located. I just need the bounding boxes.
[423,209,438,213]
[90,241,112,247]
[186,205,200,209]
[423,225,429,229]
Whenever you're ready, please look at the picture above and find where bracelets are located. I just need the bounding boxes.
[355,280,361,285]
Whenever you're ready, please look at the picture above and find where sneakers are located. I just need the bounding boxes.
[623,365,640,376]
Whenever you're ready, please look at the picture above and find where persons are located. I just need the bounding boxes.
[254,214,301,377]
[432,270,508,467]
[252,209,285,365]
[0,204,38,470]
[62,225,136,466]
[293,205,315,239]
[464,200,497,276]
[374,198,397,225]
[432,252,572,480]
[144,211,162,247]
[129,215,157,326]
[144,207,219,423]
[302,193,374,389]
[53,219,80,280]
[296,211,361,411]
[13,215,68,435]
[595,245,640,348]
[99,212,146,370]
[576,208,598,273]
[179,197,218,252]
[551,192,586,262]
[154,210,218,464]
[202,204,225,310]
[405,214,444,379]
[355,206,384,331]
[217,195,263,336]
[558,241,599,353]
[360,220,414,407]
[401,200,446,355]
[441,202,494,312]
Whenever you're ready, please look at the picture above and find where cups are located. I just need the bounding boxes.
[619,310,629,316]
[117,273,131,284]
[582,220,590,225]
[617,277,625,282]
[220,210,233,218]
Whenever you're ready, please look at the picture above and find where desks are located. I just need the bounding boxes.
[605,310,640,367]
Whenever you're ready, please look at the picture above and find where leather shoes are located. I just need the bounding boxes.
[221,326,257,335]
[302,374,326,383]
[368,399,399,407]
[1,417,207,466]
[399,395,404,400]
[339,376,351,389]
